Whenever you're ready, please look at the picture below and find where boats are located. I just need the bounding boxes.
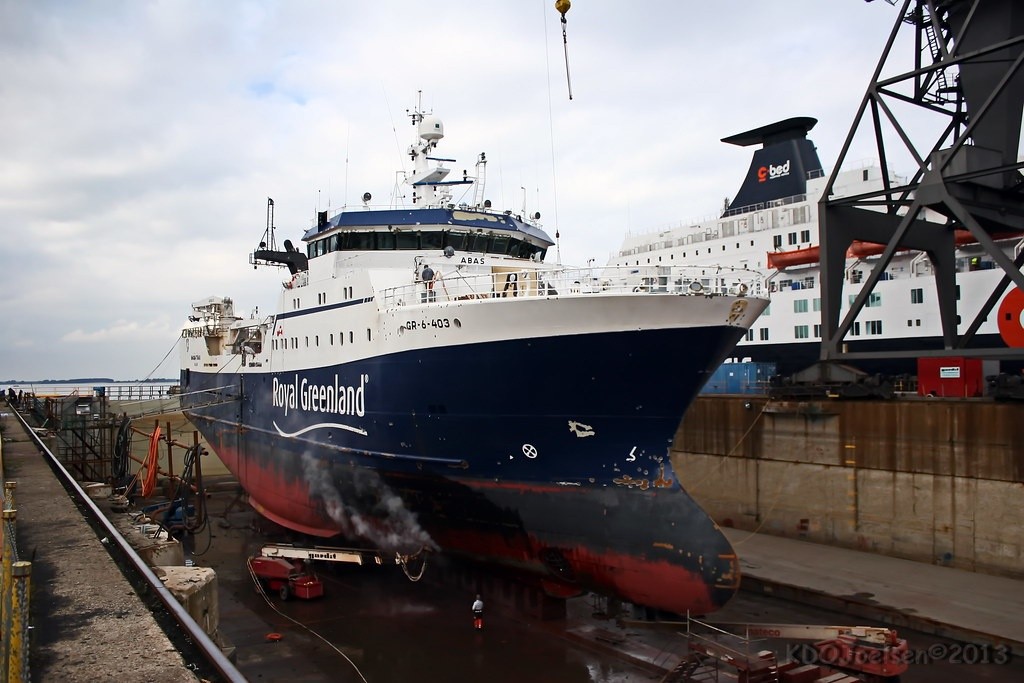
[597,1,1024,389]
[179,88,771,618]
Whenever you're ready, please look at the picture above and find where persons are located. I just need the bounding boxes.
[471,594,483,629]
[4,387,19,407]
[18,390,23,403]
[422,264,435,291]
